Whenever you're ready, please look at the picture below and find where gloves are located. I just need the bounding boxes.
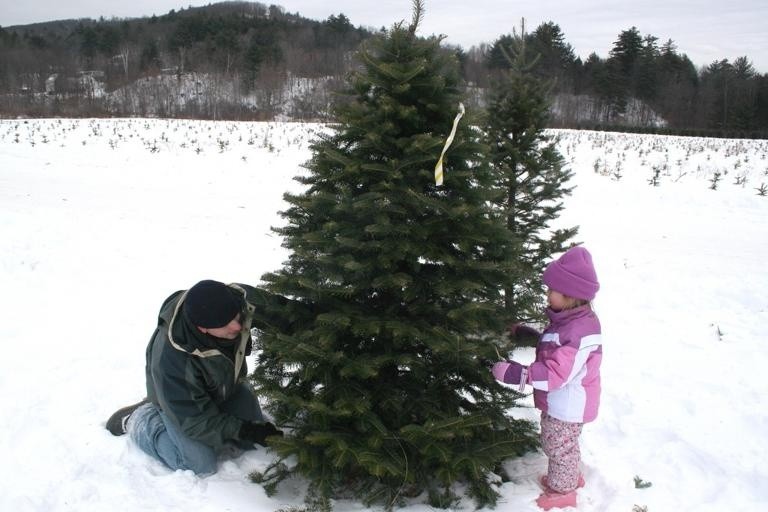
[237,418,282,444]
[490,359,528,384]
[508,323,535,341]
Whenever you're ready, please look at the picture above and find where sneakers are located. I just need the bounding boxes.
[528,489,578,511]
[106,399,148,435]
[533,471,585,488]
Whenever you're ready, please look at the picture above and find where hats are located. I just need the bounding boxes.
[187,280,242,329]
[543,246,601,303]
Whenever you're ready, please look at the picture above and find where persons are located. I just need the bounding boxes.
[105,279,305,480]
[492,244,603,512]
